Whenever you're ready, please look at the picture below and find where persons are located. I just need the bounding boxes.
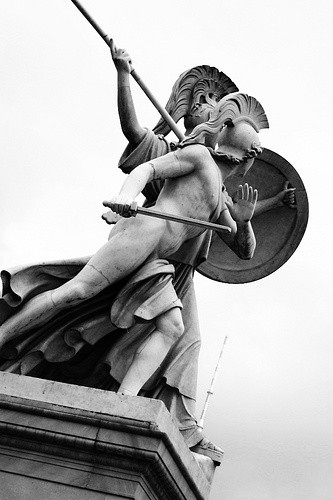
[1,88,269,397]
[2,38,298,468]
